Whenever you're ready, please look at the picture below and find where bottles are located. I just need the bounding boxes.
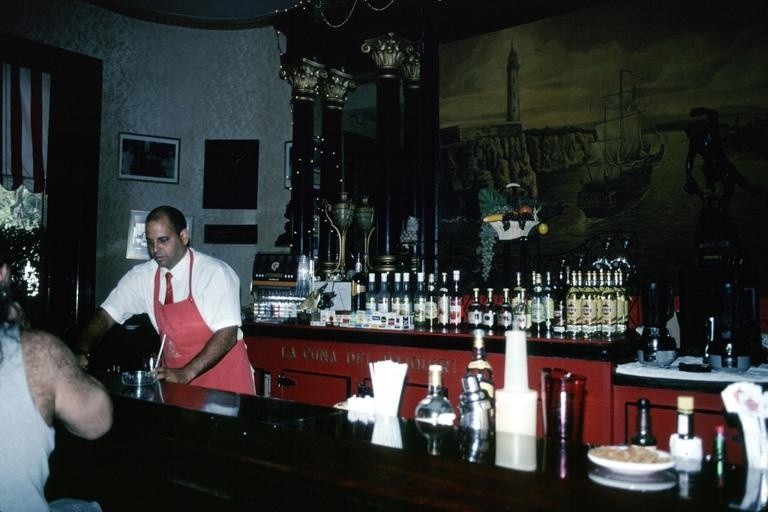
[495,434,536,475]
[673,471,702,502]
[706,426,736,462]
[493,328,537,432]
[630,394,701,462]
[411,334,492,425]
[410,426,492,465]
[331,191,376,229]
[706,463,736,495]
[347,250,633,340]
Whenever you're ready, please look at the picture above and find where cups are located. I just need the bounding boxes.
[538,366,587,443]
[364,359,408,413]
[141,352,165,369]
[542,443,581,481]
[366,412,405,450]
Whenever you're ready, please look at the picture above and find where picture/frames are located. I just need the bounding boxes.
[116,131,180,184]
[125,210,194,261]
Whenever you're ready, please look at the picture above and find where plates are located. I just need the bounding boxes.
[585,469,680,495]
[585,443,679,472]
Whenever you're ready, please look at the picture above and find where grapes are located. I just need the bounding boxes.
[502,214,533,231]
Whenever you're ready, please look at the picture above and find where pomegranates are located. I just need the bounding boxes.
[519,204,533,214]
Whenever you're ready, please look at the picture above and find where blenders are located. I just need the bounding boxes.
[638,279,679,365]
[704,279,760,373]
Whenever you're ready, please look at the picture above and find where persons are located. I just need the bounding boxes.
[72,206,256,396]
[0,243,114,512]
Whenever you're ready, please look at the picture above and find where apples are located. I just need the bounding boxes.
[538,223,550,235]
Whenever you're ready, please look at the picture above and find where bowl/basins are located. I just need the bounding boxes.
[124,387,158,400]
[122,370,158,386]
[489,219,541,241]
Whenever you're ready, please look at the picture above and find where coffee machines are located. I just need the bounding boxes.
[251,251,314,320]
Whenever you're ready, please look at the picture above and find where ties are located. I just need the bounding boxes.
[164,272,174,305]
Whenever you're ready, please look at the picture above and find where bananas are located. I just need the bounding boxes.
[483,214,503,223]
